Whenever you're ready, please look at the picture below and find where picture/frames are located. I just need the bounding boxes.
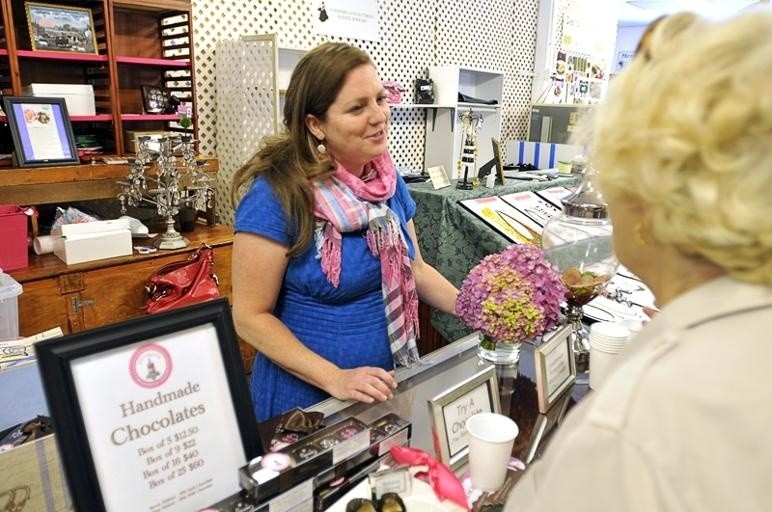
[428,166,451,190]
[25,1,99,55]
[3,96,80,168]
[34,298,275,512]
[534,325,577,414]
[457,196,543,258]
[429,365,519,470]
[499,191,558,229]
[479,138,505,183]
[535,186,569,214]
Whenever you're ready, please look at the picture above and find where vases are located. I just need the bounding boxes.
[477,346,521,416]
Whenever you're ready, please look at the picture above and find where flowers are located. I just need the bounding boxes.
[452,242,569,351]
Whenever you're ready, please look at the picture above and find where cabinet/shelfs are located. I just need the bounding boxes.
[216,31,504,179]
[258,261,662,512]
[0,0,259,372]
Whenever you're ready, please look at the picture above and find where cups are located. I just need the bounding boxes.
[588,321,632,391]
[465,412,520,492]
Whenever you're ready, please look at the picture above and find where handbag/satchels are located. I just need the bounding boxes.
[144,242,221,315]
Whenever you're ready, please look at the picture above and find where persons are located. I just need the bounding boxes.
[232,42,461,424]
[501,0,770,510]
